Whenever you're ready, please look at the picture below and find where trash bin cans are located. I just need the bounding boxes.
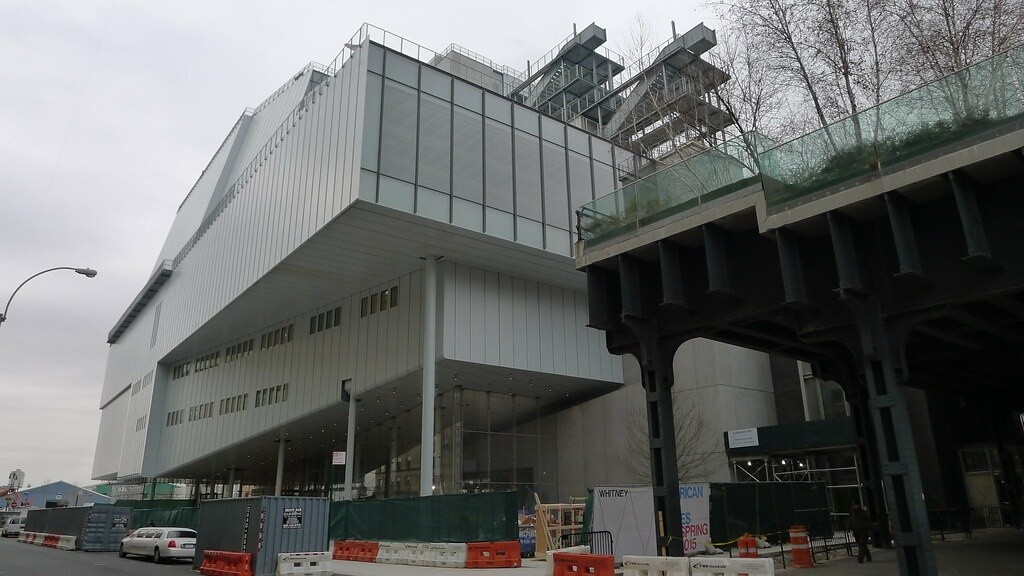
[518,525,536,558]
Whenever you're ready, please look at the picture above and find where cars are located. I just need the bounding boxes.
[119,526,197,562]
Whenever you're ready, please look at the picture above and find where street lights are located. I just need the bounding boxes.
[0,265,97,324]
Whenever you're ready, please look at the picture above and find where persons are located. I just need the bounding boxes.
[847,500,873,563]
[958,505,972,539]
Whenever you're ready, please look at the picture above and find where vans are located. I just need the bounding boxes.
[2,516,27,538]
